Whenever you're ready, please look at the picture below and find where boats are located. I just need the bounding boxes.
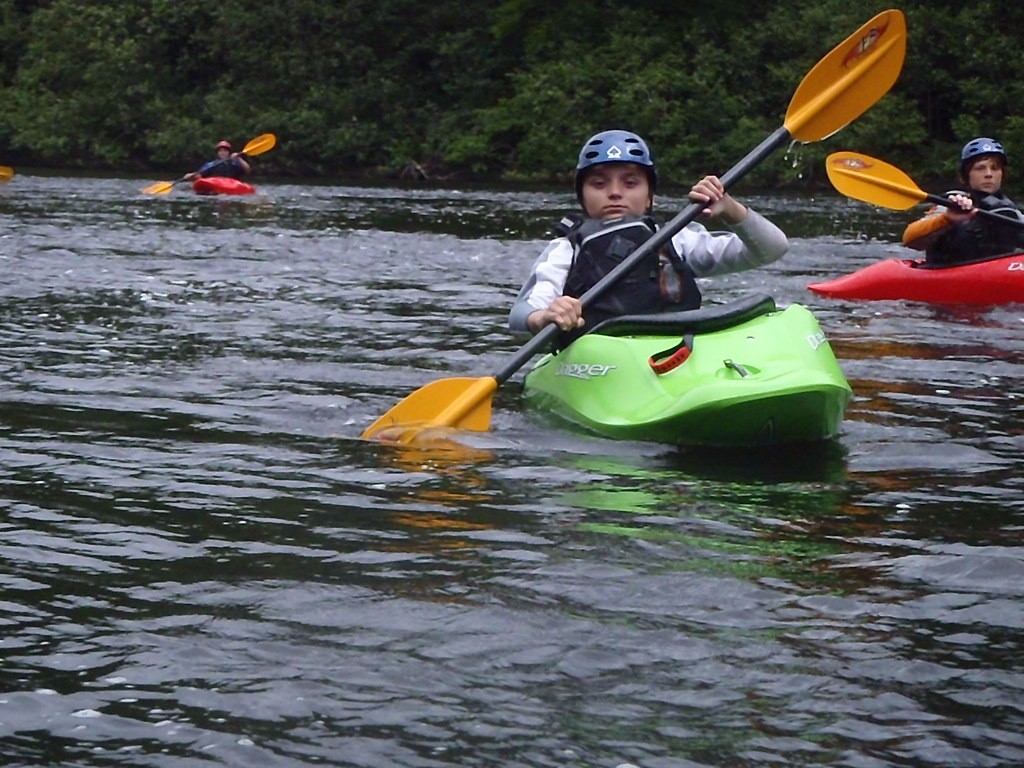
[193,175,256,197]
[805,258,1024,309]
[522,294,855,448]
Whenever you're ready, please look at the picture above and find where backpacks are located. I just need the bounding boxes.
[556,211,701,346]
[963,191,1020,257]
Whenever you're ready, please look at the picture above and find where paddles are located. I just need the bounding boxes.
[142,133,277,196]
[358,7,910,442]
[824,150,1024,229]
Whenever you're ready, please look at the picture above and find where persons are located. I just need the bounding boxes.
[902,137,1024,268]
[509,130,790,351]
[184,140,251,180]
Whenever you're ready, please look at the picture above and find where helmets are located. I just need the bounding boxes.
[215,141,232,151]
[960,137,1005,161]
[576,130,654,169]
